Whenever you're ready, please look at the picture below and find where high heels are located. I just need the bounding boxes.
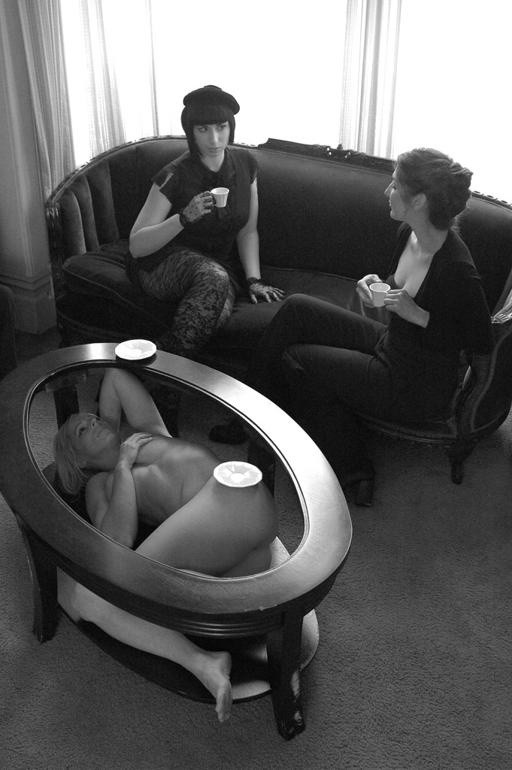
[323,450,378,507]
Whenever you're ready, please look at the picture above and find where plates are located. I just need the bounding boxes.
[213,460,265,490]
[115,338,157,362]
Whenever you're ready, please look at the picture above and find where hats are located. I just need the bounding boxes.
[183,84,241,113]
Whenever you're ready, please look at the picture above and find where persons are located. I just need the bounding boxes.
[54,368,276,723]
[210,147,489,509]
[127,84,285,438]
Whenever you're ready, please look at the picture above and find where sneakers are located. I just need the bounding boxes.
[160,407,181,439]
[207,416,251,447]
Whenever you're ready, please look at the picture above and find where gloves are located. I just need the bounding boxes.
[240,277,287,304]
[176,191,216,228]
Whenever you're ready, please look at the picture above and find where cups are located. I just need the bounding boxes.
[210,187,230,208]
[370,283,390,308]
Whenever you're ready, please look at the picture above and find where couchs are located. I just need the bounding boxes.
[40,129,512,487]
